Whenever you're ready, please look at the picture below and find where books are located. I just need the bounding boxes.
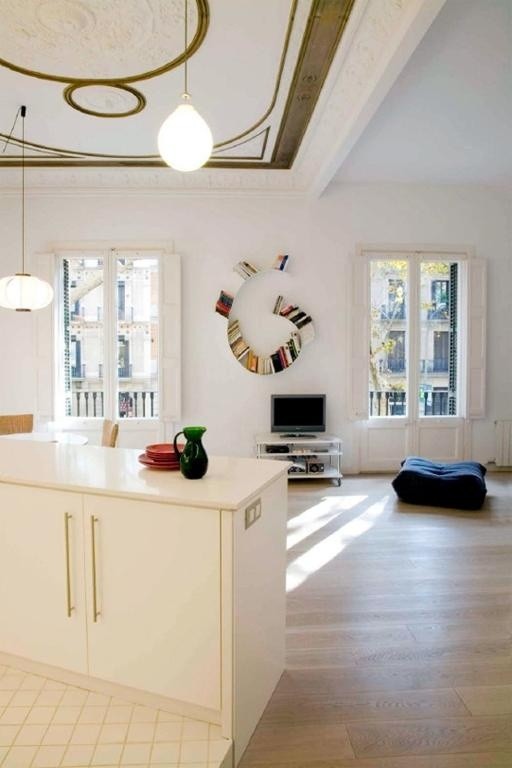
[215,254,312,375]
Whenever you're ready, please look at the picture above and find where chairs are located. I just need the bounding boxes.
[102,420,119,448]
[0,414,34,434]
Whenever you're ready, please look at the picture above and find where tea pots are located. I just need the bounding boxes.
[171,427,211,479]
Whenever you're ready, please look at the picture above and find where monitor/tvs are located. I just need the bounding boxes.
[269,393,327,439]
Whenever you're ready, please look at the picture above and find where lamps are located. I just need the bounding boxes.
[0,106,53,312]
[158,0,213,172]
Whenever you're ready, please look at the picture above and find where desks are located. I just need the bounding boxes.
[7,433,89,444]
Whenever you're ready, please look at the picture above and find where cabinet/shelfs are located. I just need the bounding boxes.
[256,436,343,486]
[0,445,294,768]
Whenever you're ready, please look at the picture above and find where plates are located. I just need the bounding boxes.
[139,443,187,471]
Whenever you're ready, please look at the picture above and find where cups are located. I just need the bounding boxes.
[46,420,60,442]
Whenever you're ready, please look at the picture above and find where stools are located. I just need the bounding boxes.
[392,458,487,510]
